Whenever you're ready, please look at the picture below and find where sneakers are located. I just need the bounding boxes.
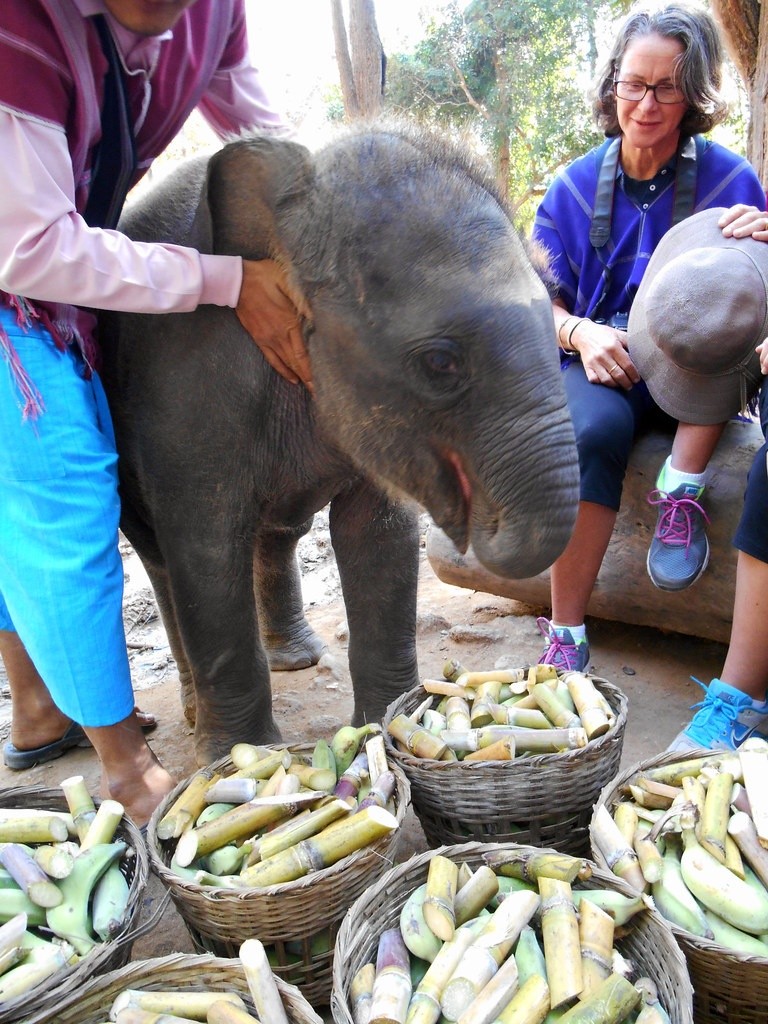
[533,618,592,670]
[659,675,768,756]
[647,466,713,594]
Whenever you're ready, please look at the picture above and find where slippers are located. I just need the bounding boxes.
[3,707,157,772]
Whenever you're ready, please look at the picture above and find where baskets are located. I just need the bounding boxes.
[330,841,694,1024]
[0,786,171,1024]
[380,667,627,860]
[589,748,768,1024]
[21,954,324,1024]
[148,741,431,1011]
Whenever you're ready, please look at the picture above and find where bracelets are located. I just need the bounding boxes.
[557,315,589,357]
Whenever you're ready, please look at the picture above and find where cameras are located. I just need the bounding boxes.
[593,311,628,332]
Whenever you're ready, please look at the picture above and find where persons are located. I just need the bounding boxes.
[667,337,768,753]
[494,1,768,675]
[0,0,314,843]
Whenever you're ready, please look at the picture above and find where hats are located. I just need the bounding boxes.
[628,206,768,425]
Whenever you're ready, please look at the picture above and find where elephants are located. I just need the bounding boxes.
[89,124,585,772]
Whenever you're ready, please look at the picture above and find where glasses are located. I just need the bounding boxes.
[612,80,687,106]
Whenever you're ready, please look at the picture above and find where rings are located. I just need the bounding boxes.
[608,364,618,374]
